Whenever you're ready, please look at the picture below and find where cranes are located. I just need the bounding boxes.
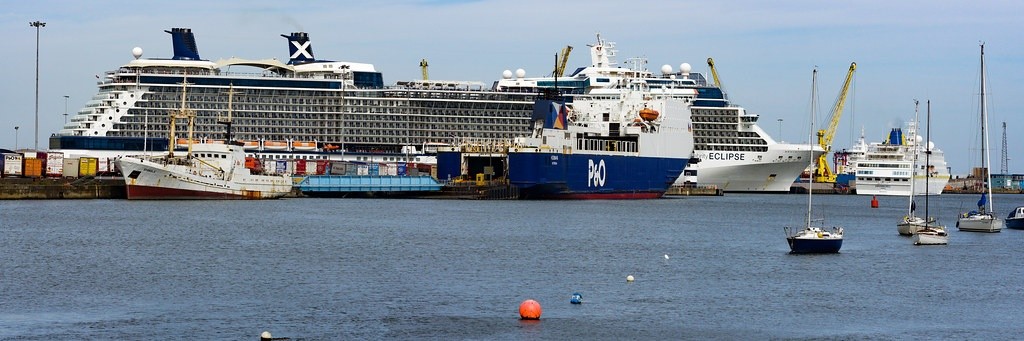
[1000,122,1011,189]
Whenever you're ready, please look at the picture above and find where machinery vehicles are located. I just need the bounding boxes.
[808,62,858,183]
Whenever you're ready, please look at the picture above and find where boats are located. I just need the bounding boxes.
[854,118,953,198]
[264,141,288,152]
[292,141,316,154]
[504,33,698,200]
[111,69,299,201]
[1005,206,1024,230]
[639,107,659,122]
[242,141,259,150]
[206,140,226,145]
[176,138,200,151]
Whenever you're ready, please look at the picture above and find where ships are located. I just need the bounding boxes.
[49,26,827,195]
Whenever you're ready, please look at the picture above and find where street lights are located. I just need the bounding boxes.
[63,95,70,124]
[29,20,45,153]
[14,126,20,151]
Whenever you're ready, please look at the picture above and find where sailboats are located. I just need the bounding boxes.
[784,69,844,256]
[955,40,1004,233]
[895,99,934,237]
[910,99,950,246]
